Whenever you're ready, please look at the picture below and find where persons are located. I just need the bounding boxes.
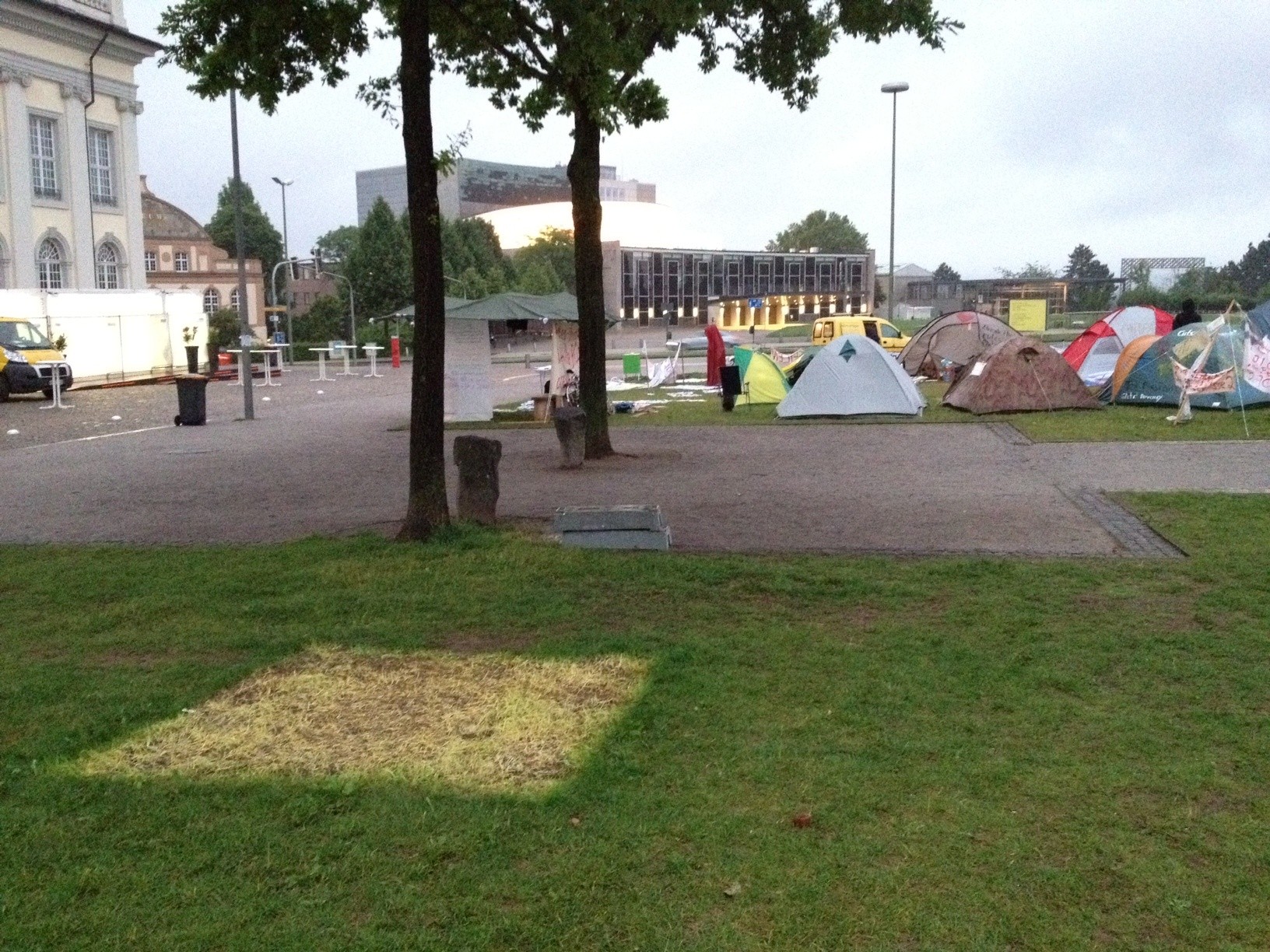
[1172,298,1202,331]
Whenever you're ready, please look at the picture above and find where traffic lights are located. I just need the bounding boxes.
[310,247,321,268]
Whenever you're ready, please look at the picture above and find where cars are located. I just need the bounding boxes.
[665,325,741,353]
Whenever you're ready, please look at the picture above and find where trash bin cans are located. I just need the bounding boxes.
[174,373,209,426]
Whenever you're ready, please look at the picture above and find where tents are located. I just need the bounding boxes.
[775,334,926,416]
[733,346,791,406]
[704,324,726,386]
[1061,304,1175,386]
[895,310,1023,379]
[1239,301,1270,338]
[1094,320,1270,412]
[941,337,1107,417]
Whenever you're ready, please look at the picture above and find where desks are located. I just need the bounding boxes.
[362,346,384,378]
[335,344,359,376]
[308,348,336,381]
[254,351,282,387]
[271,343,292,372]
[223,348,255,387]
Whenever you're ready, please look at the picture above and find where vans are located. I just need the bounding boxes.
[812,312,914,353]
[0,317,73,403]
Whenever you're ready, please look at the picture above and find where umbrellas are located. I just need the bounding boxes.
[378,291,628,331]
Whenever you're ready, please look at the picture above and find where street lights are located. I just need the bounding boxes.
[880,80,909,323]
[270,176,294,368]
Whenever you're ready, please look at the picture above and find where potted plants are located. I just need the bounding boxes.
[183,326,197,373]
